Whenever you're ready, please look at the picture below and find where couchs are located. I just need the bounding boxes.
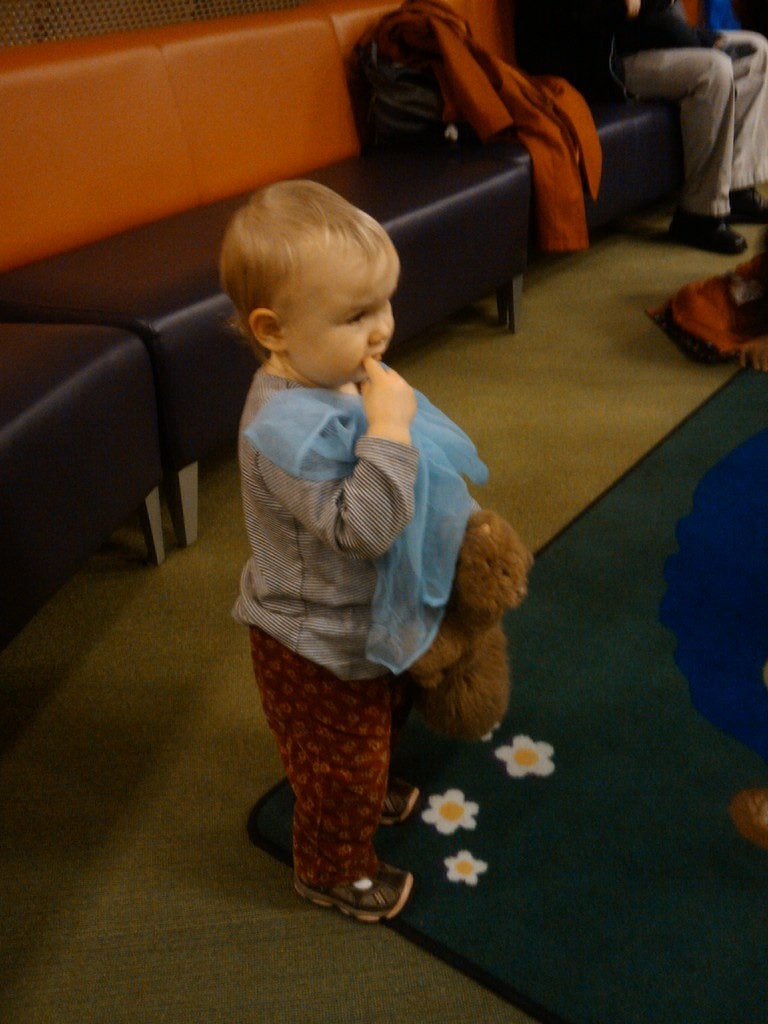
[302,0,754,327]
[0,320,168,657]
[0,4,535,548]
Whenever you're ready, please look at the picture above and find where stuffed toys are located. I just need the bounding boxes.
[400,508,534,739]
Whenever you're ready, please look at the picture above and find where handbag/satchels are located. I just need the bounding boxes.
[364,37,474,151]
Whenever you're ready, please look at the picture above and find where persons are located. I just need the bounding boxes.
[224,178,419,922]
[518,0,768,253]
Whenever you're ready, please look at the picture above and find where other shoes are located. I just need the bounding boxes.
[727,184,768,227]
[670,208,749,254]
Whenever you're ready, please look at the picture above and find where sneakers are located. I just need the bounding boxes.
[377,779,420,826]
[294,859,413,921]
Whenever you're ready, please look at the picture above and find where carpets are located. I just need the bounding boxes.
[246,362,768,1024]
